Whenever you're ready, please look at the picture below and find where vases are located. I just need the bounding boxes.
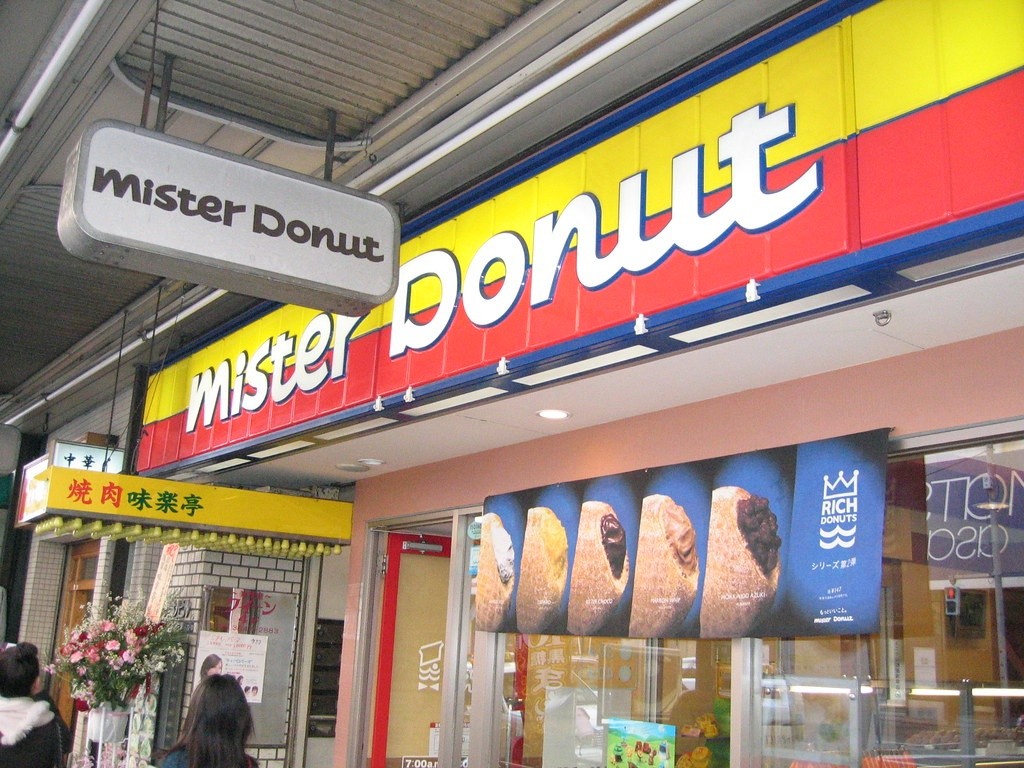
[88,701,129,743]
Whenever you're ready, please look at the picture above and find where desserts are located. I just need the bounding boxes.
[514,506,569,634]
[566,499,630,636]
[629,493,699,637]
[700,483,782,639]
[474,512,516,631]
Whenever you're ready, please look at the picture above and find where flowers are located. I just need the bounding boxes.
[40,576,185,712]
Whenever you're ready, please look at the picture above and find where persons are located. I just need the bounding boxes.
[159,674,259,768]
[237,676,243,685]
[0,642,73,768]
[244,686,251,693]
[252,686,258,696]
[200,654,222,680]
